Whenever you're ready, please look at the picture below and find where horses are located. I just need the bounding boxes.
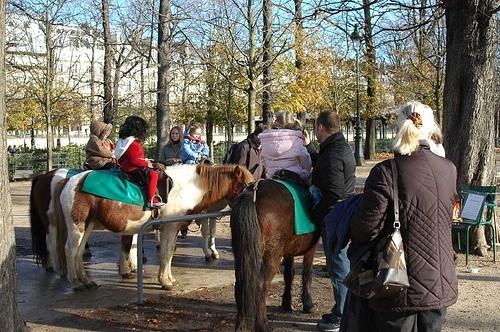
[29,160,259,292]
[230,177,321,332]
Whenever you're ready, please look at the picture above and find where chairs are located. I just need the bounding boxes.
[451,183,497,266]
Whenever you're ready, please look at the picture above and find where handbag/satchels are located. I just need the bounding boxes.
[342,230,410,298]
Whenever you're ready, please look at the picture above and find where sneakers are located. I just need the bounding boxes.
[322,312,337,322]
[322,323,340,331]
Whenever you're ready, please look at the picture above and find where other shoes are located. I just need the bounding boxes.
[147,202,166,208]
[182,233,187,238]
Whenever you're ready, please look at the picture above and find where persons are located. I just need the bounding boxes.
[259,111,313,186]
[85,120,119,170]
[349,101,457,332]
[162,126,182,166]
[114,116,165,207]
[302,110,357,331]
[182,124,211,165]
[230,124,271,181]
